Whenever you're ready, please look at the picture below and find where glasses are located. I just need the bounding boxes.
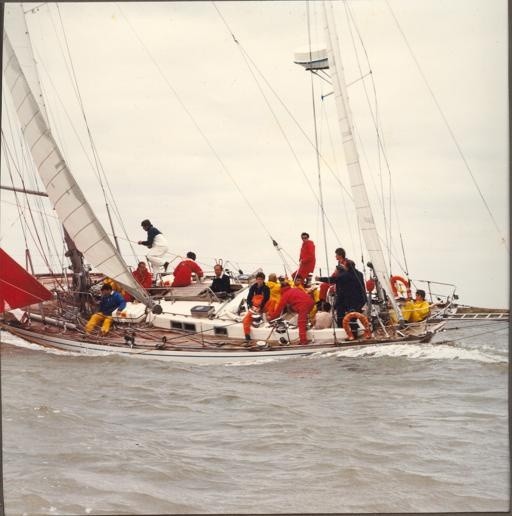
[301,236,308,239]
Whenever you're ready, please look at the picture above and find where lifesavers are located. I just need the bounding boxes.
[344,312,371,340]
[390,276,413,301]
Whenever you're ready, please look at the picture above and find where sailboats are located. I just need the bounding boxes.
[0,1,506,365]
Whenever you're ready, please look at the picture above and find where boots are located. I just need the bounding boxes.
[246,335,250,340]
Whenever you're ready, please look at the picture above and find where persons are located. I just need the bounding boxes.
[390,289,431,324]
[171,252,204,287]
[330,248,348,277]
[132,262,153,289]
[267,281,315,345]
[243,272,270,340]
[209,263,232,294]
[287,275,307,293]
[315,260,368,341]
[265,273,287,312]
[292,232,316,281]
[332,264,346,328]
[84,284,126,337]
[137,219,170,275]
